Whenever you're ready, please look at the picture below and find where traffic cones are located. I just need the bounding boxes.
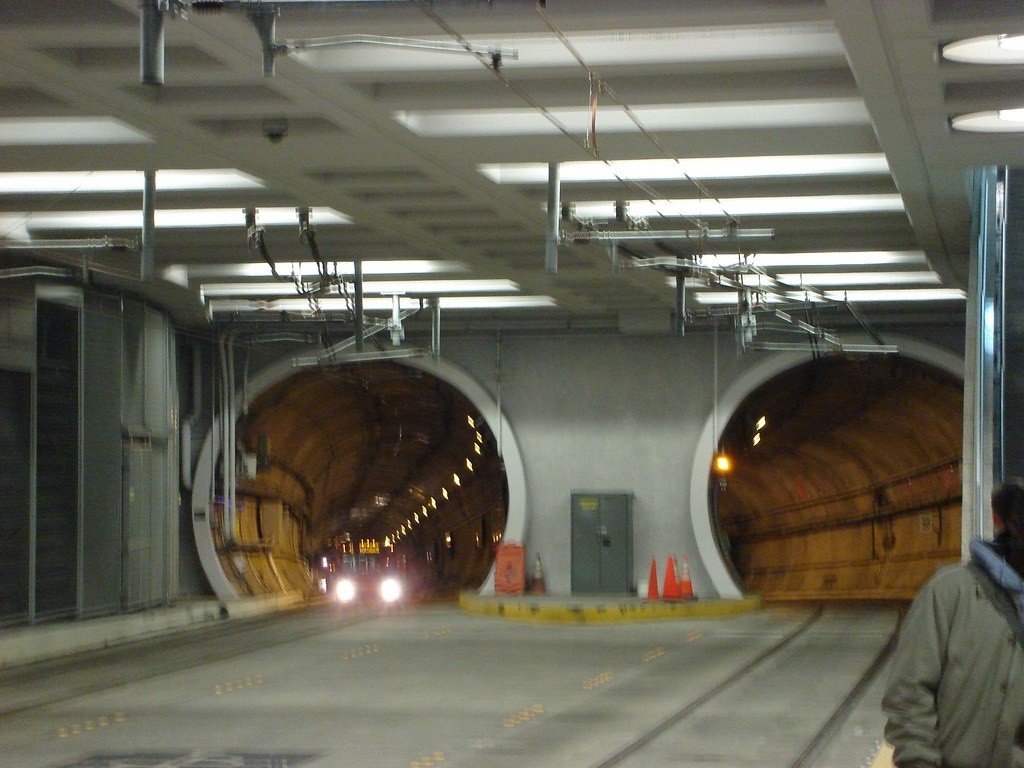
[670,554,684,602]
[664,553,682,603]
[641,557,662,604]
[530,553,547,597]
[679,552,699,600]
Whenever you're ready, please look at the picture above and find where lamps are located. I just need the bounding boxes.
[952,109,1024,133]
[942,34,1024,65]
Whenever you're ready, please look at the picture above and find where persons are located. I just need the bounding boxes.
[878,475,1024,768]
